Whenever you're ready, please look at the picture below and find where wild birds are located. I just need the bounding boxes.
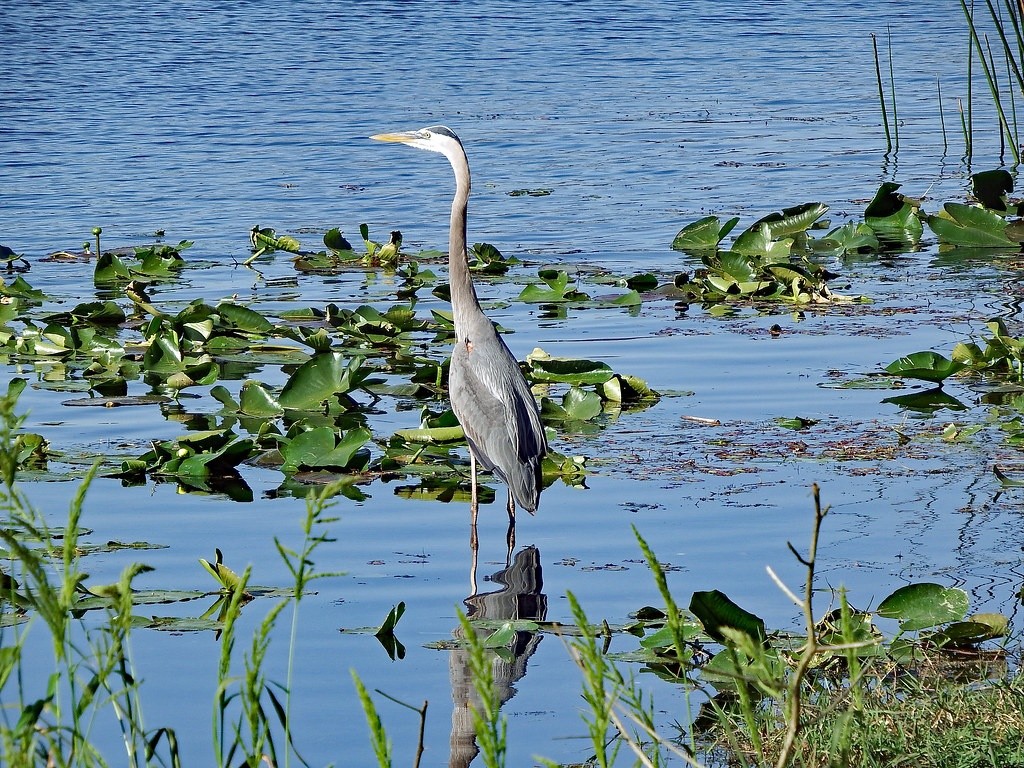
[365,122,551,526]
[443,524,551,767]
